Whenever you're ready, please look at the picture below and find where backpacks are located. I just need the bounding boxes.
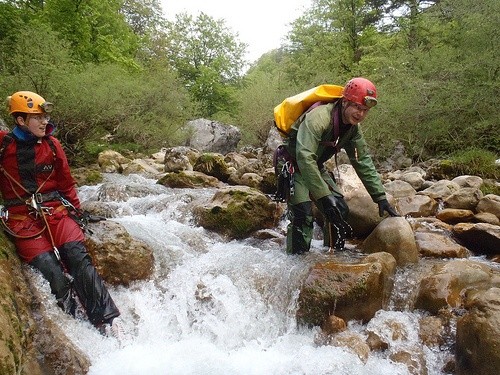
[272,84,344,154]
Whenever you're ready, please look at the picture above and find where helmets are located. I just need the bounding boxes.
[7,91,46,115]
[342,77,378,108]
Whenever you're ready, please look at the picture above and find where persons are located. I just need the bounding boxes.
[0,91,131,349]
[273,77,399,255]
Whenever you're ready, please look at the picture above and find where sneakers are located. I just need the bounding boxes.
[102,317,132,350]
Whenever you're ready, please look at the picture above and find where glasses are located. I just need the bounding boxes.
[27,114,50,122]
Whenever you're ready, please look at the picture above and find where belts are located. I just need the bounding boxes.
[5,192,59,206]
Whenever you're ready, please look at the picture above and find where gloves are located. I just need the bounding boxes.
[378,199,400,219]
[80,212,107,222]
[316,195,345,224]
